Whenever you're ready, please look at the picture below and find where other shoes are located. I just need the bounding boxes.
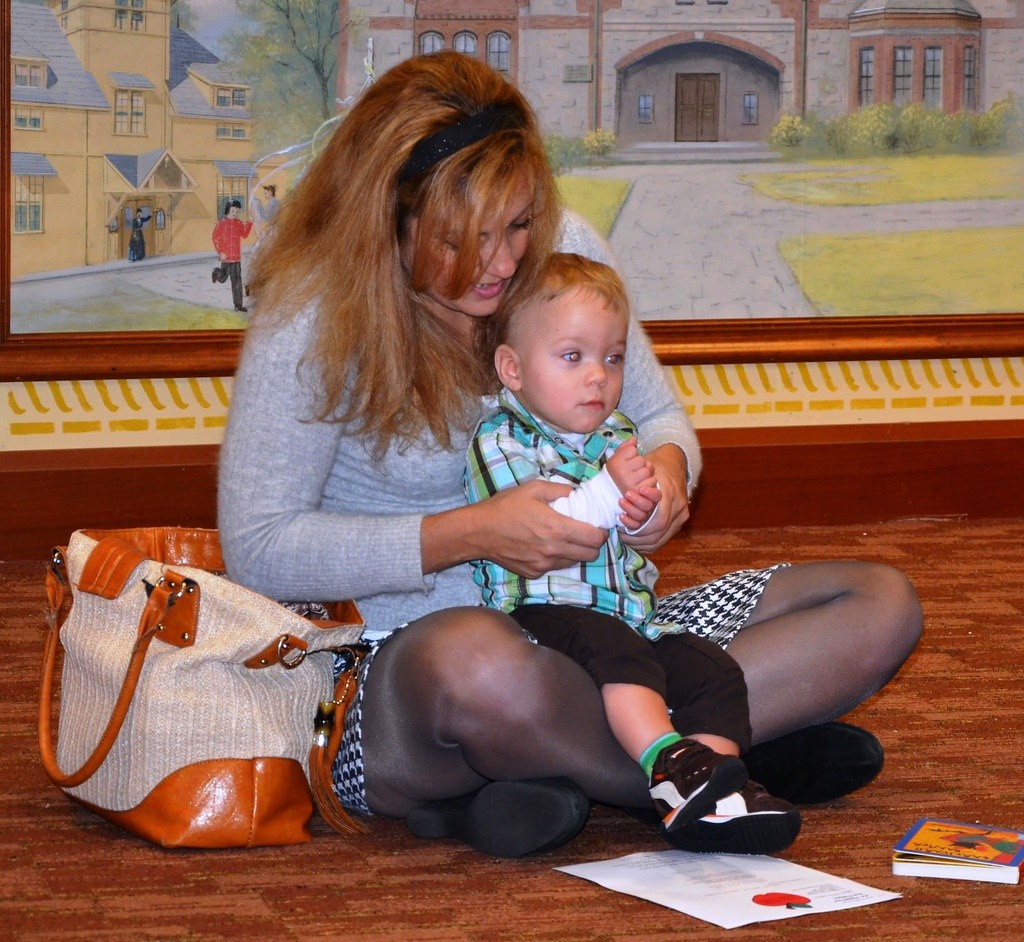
[739,721,884,805]
[403,776,594,859]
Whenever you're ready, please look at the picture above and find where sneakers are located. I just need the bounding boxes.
[665,780,802,855]
[647,739,745,833]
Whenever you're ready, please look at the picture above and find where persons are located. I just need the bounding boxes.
[464,253,802,855]
[216,52,925,852]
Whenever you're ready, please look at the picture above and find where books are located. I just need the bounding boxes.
[891,818,1024,884]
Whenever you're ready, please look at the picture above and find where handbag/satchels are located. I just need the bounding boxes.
[39,523,367,844]
